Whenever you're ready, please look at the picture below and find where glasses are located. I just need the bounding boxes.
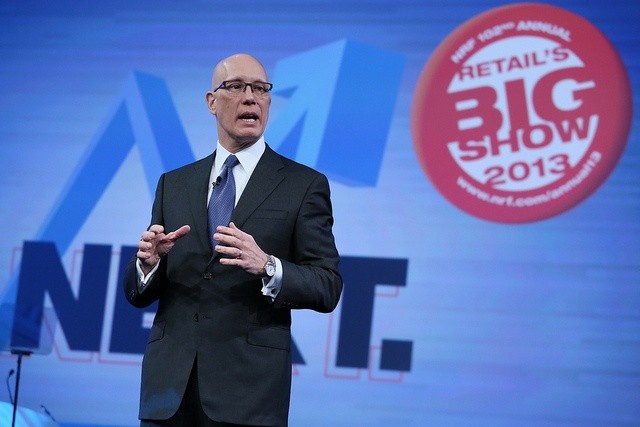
[213,79,273,92]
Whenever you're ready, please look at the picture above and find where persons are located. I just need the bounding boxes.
[125,53,343,427]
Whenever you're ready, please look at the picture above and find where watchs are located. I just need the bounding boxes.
[260,255,277,277]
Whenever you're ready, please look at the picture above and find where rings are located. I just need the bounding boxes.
[236,251,243,259]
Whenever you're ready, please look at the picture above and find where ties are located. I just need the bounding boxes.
[207,154,241,251]
